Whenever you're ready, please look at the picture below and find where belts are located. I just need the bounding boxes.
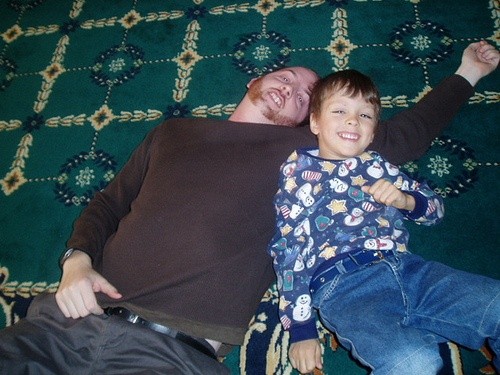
[102,306,216,360]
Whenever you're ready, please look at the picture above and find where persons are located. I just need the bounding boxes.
[1,41,500,375]
[269,70,500,375]
